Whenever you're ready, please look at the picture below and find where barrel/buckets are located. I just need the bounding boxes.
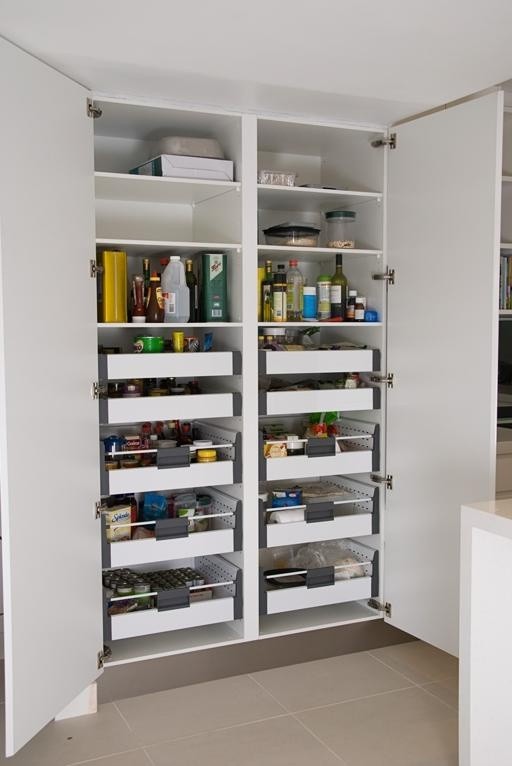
[160,256,190,323]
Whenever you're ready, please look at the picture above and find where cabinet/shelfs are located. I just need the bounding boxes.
[1,84,512,758]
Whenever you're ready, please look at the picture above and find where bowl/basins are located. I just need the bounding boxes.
[133,336,164,352]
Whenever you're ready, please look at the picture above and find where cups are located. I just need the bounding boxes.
[172,332,184,352]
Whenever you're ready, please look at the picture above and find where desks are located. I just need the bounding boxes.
[458,499,511,766]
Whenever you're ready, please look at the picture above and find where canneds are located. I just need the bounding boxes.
[197,449,216,463]
[105,459,139,471]
[104,568,205,611]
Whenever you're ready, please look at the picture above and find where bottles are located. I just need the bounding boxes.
[185,260,199,322]
[192,494,212,533]
[261,253,364,322]
[104,435,120,469]
[140,258,165,323]
[142,421,217,466]
[325,209,357,249]
